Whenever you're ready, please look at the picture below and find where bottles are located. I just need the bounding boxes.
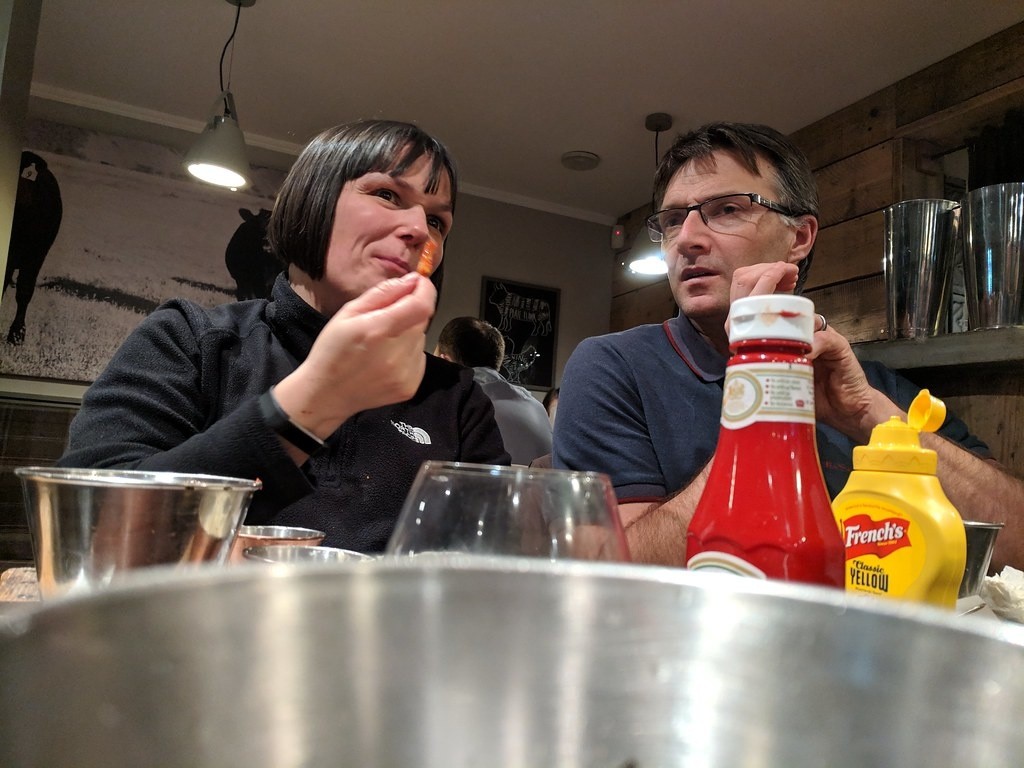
[686,295,846,594]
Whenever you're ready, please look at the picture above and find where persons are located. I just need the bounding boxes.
[49,118,511,607]
[427,315,555,470]
[543,122,1024,621]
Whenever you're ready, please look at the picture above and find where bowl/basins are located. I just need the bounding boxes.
[16,464,262,603]
[2,550,1020,767]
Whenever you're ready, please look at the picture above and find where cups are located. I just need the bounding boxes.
[382,460,631,561]
[232,523,325,565]
[957,519,1004,601]
[244,545,374,566]
[884,198,960,338]
[960,183,1024,333]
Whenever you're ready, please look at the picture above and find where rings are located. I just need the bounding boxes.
[814,310,829,335]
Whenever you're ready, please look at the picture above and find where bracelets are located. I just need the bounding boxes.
[259,384,331,461]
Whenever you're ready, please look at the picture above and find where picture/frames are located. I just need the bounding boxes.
[478,274,562,393]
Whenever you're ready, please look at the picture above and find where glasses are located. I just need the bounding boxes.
[645,192,801,242]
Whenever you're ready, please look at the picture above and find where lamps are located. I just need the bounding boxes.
[181,0,259,191]
[620,112,673,279]
